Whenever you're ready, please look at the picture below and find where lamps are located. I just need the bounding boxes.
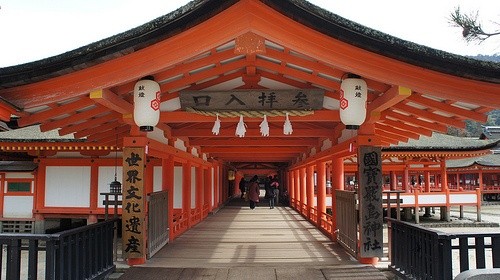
[355,180,359,193]
[109,128,121,194]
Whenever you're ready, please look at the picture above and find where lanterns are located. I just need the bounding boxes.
[340,74,367,130]
[134,75,160,131]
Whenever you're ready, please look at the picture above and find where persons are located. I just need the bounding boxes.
[273,175,283,203]
[250,174,258,183]
[282,188,289,206]
[269,176,272,179]
[419,177,421,185]
[248,177,259,210]
[271,179,279,189]
[411,177,415,185]
[239,177,246,198]
[386,177,389,183]
[265,177,274,209]
[347,177,352,184]
